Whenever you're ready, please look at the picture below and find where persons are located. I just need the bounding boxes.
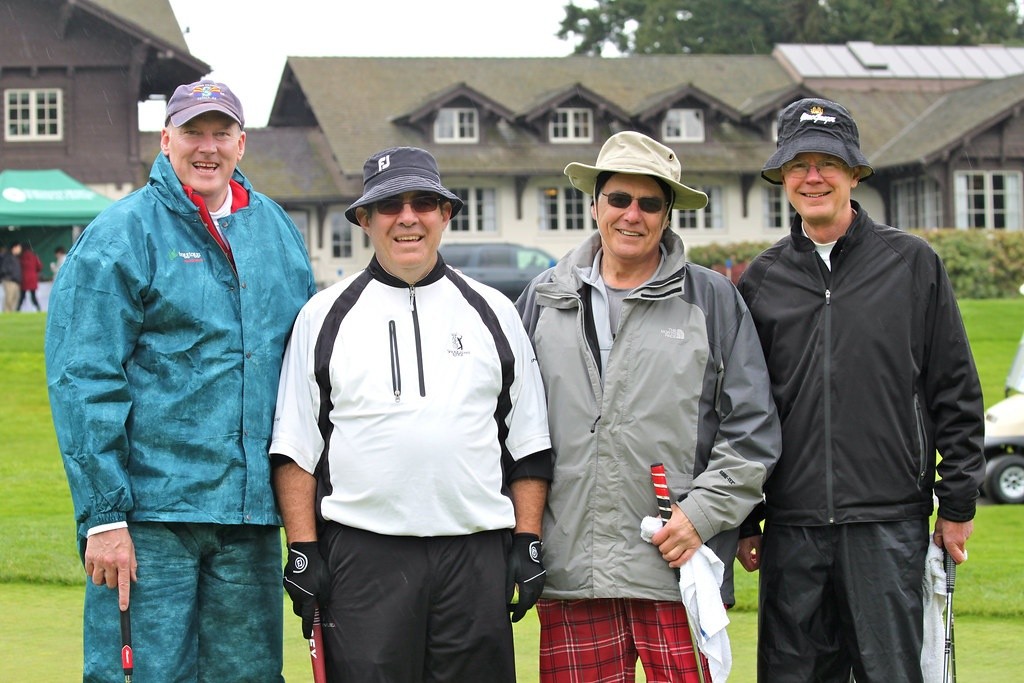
[0,243,66,313]
[510,133,783,683]
[45,80,317,683]
[732,98,986,683]
[267,145,554,683]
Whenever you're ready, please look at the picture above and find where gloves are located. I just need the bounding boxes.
[505,532,547,623]
[282,540,330,640]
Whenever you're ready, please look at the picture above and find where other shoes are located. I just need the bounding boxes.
[37,305,41,311]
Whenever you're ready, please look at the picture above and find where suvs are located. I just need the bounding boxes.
[439,243,556,302]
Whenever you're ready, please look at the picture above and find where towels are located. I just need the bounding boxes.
[916,532,971,682]
[637,510,733,683]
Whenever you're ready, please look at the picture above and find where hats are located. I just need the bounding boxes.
[164,80,246,132]
[344,146,465,226]
[761,98,874,185]
[55,247,66,253]
[562,131,708,210]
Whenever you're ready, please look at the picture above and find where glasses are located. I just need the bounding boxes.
[365,196,441,215]
[602,192,669,214]
[782,161,847,178]
